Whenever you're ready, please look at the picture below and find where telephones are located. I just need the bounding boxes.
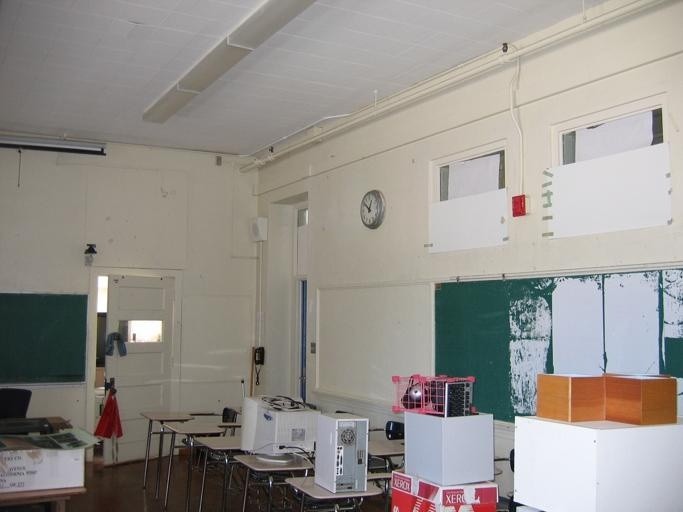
[254,347,264,365]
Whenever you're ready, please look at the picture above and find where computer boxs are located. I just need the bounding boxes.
[314,411,368,494]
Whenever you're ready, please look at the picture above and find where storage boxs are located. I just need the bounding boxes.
[536,373,604,425]
[516,505,546,512]
[513,414,682,512]
[0,432,86,494]
[604,373,678,427]
[404,412,495,484]
[390,469,498,512]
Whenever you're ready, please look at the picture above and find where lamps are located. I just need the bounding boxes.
[140,2,318,128]
[0,135,107,155]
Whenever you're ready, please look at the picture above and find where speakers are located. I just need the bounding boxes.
[249,216,267,243]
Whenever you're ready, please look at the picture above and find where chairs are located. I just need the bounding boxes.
[0,387,32,418]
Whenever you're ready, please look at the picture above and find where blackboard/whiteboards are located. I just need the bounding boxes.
[314,279,436,408]
[0,294,88,384]
[433,262,683,423]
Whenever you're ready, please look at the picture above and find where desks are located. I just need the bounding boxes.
[0,417,87,512]
[139,408,405,512]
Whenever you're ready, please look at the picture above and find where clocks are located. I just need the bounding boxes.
[361,191,386,229]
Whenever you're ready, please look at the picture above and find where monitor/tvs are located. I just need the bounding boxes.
[239,395,320,465]
